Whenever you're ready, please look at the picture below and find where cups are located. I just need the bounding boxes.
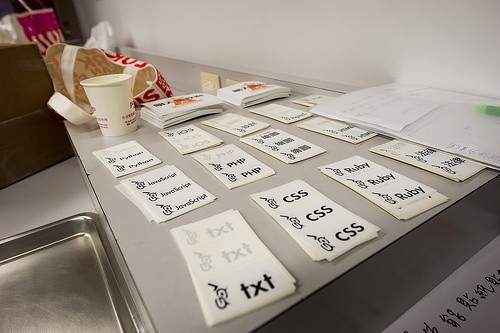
[78,73,138,137]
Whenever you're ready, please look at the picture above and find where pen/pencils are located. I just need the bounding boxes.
[476,105,500,116]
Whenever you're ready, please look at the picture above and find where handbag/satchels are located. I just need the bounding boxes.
[2,9,65,61]
[45,43,174,125]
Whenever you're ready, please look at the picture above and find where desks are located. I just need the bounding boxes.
[62,46,500,331]
